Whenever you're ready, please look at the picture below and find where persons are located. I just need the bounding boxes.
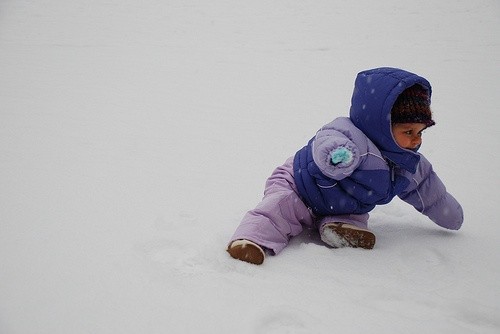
[226,67,464,266]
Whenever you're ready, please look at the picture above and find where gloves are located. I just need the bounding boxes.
[332,146,353,165]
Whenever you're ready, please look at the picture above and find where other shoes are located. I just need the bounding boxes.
[320,222,377,249]
[228,238,265,265]
